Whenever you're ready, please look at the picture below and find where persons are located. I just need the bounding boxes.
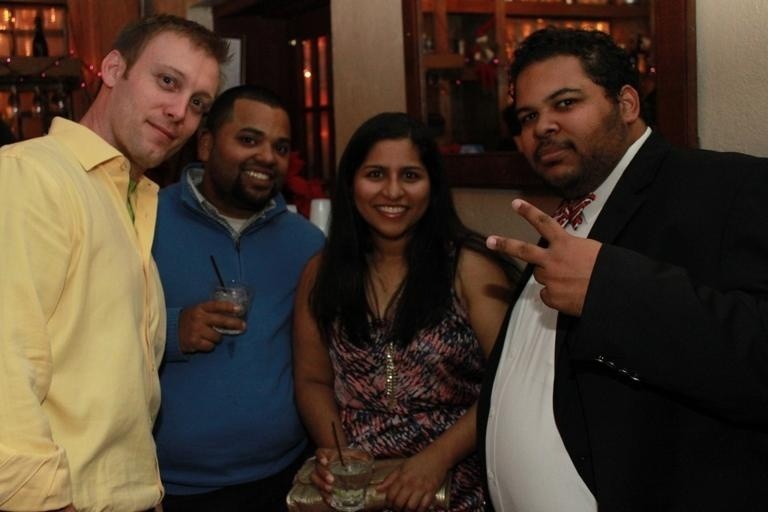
[2,12,237,511]
[282,110,524,511]
[476,27,768,512]
[155,84,328,511]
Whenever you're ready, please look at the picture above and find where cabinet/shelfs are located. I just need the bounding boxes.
[401,0,700,191]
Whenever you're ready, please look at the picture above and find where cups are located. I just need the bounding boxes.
[210,280,251,335]
[325,446,375,512]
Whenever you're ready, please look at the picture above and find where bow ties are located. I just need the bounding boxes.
[551,194,597,230]
